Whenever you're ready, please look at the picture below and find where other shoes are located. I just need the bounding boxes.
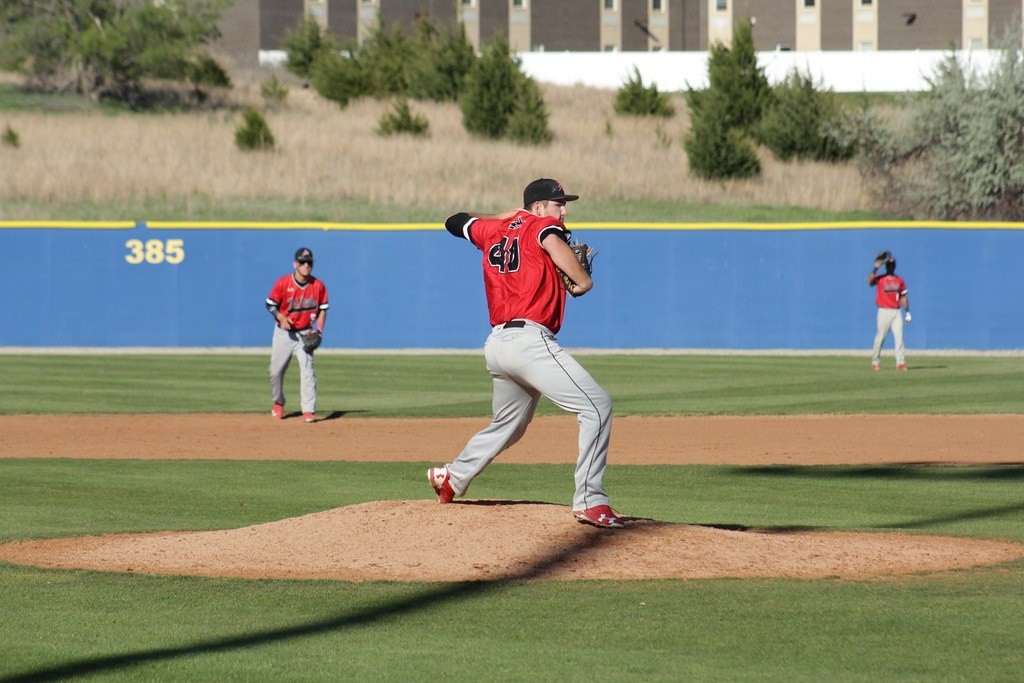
[873,362,880,370]
[898,364,906,371]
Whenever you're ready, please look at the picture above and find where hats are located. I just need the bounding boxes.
[295,248,314,262]
[524,178,579,207]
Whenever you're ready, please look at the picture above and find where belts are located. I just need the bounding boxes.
[503,321,526,329]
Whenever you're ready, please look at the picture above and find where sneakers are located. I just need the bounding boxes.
[303,413,315,422]
[272,403,283,419]
[574,504,624,527]
[428,467,455,503]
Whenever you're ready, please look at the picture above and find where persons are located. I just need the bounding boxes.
[427,179,662,528]
[264,248,329,422]
[866,250,912,370]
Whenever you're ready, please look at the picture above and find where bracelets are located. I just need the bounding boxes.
[906,309,909,312]
[317,322,324,332]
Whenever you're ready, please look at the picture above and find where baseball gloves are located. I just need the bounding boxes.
[301,330,321,353]
[554,243,591,297]
[874,252,889,269]
